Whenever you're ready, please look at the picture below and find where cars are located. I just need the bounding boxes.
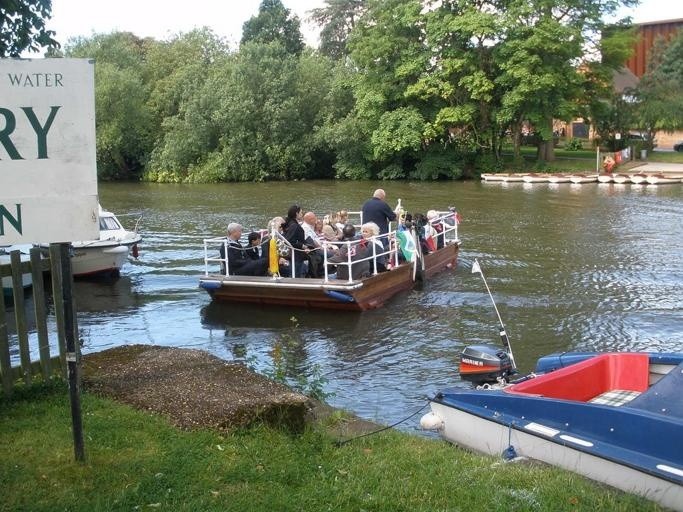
[593,132,657,151]
[673,142,682,152]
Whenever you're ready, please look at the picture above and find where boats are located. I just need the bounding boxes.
[420,258,682,511]
[0,237,53,301]
[34,190,150,293]
[198,203,461,310]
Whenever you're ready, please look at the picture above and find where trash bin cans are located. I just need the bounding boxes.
[640,150,647,162]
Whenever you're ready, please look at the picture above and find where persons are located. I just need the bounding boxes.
[218,187,444,282]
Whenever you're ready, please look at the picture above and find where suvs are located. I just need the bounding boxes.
[519,128,559,147]
